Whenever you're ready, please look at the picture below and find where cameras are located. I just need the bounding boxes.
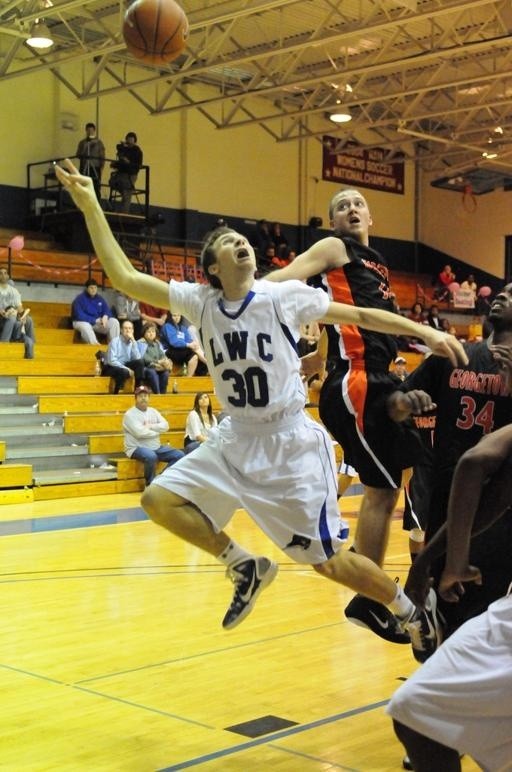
[86,135,97,141]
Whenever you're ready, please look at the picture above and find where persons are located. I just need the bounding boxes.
[76,123,106,204]
[256,188,425,645]
[53,158,439,665]
[388,280,512,656]
[71,279,210,395]
[391,356,410,381]
[1,268,34,358]
[398,264,492,357]
[109,132,142,211]
[256,218,330,387]
[386,595,510,772]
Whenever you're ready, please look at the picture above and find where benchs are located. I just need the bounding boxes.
[0,225,468,491]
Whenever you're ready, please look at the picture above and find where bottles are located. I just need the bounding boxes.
[95,362,101,377]
[448,272,455,280]
[174,379,178,394]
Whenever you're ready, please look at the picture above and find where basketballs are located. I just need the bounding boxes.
[122,0,188,66]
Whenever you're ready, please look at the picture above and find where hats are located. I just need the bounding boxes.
[135,384,150,394]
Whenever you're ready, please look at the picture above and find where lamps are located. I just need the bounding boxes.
[26,18,54,48]
[330,107,353,123]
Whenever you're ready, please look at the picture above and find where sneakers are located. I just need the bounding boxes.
[223,555,278,630]
[345,594,410,644]
[394,587,442,663]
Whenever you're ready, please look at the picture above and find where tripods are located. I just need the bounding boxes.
[113,151,145,216]
[82,141,115,212]
